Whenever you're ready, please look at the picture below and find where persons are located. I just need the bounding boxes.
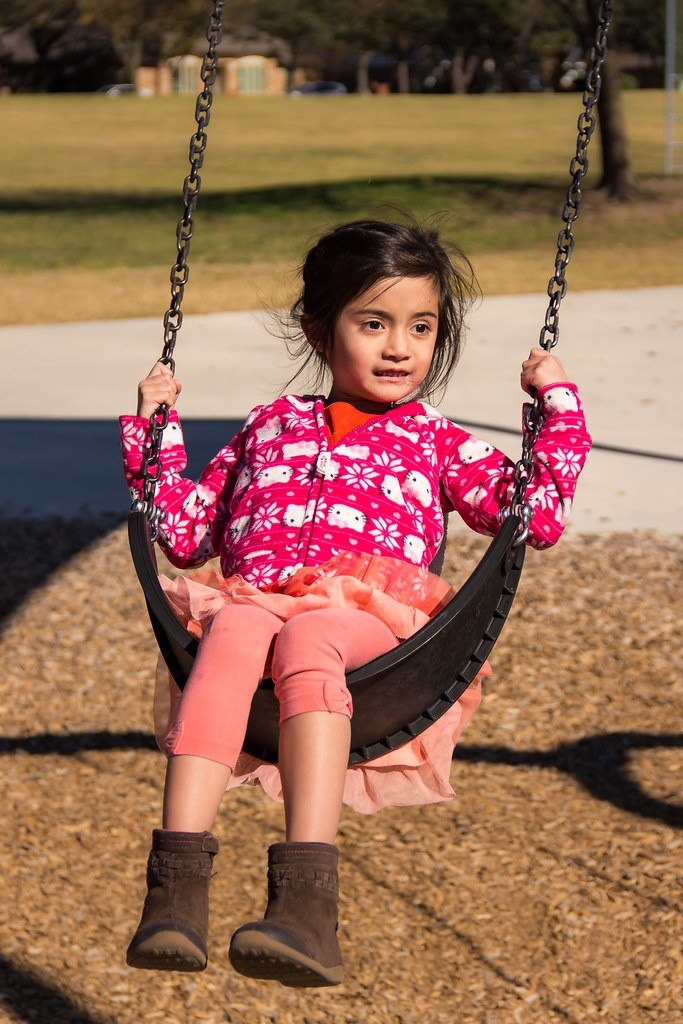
[119,217,592,987]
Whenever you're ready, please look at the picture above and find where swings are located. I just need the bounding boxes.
[122,2,623,774]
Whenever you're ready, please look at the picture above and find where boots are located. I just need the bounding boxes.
[227,841,344,988]
[126,828,218,973]
